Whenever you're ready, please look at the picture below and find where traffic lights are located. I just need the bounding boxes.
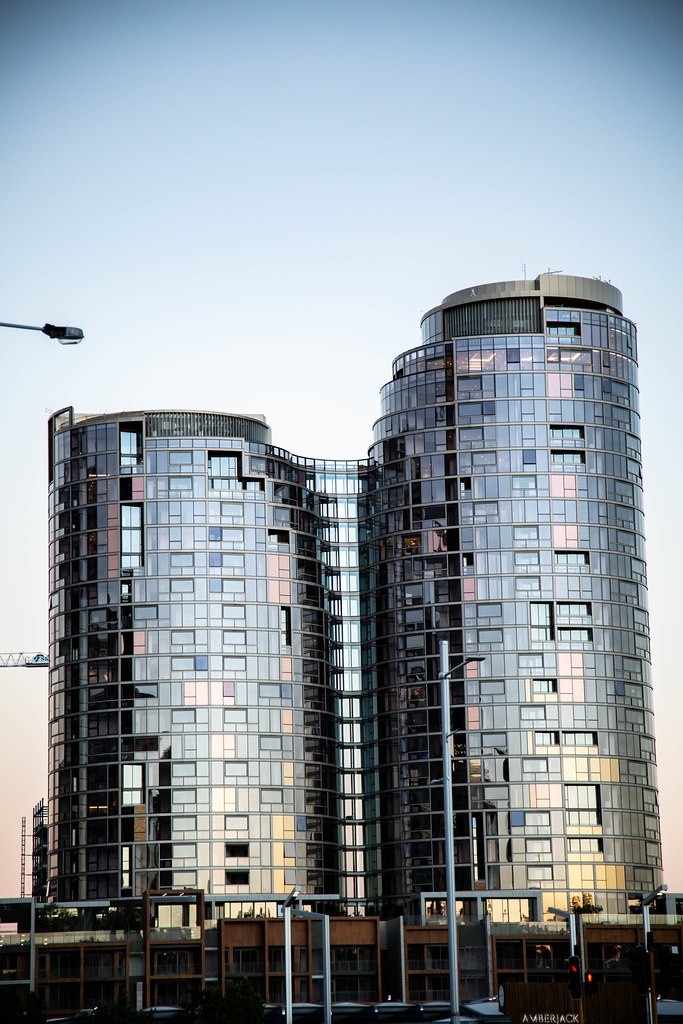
[568,955,583,1001]
[584,970,593,983]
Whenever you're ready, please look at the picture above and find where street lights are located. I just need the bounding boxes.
[436,640,486,1024]
